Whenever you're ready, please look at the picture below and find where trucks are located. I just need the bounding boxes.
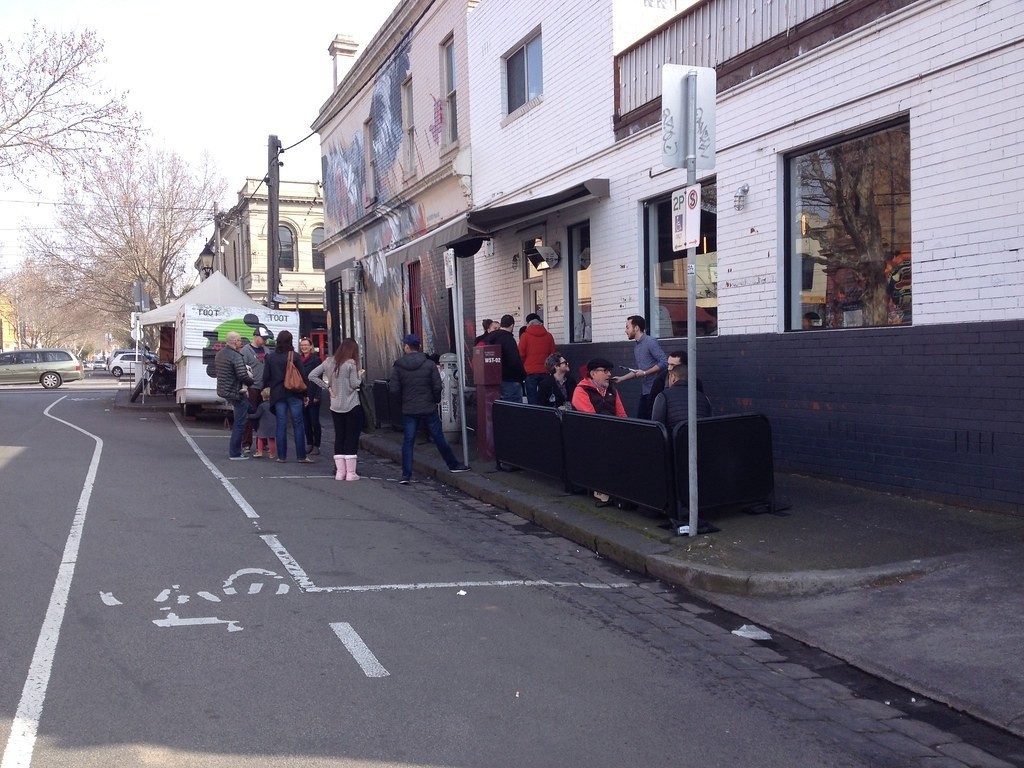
[173,303,300,416]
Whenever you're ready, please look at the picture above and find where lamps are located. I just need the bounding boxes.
[734,183,749,210]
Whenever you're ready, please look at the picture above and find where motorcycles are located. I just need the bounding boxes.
[131,346,176,402]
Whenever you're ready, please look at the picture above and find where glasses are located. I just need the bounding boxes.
[559,360,568,365]
[594,368,612,374]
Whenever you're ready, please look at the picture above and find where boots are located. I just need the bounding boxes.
[333,454,346,480]
[344,455,360,481]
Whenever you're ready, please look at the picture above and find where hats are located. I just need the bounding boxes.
[588,358,614,370]
[402,334,421,345]
[253,327,272,339]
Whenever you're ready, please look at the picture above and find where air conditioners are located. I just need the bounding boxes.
[341,268,357,290]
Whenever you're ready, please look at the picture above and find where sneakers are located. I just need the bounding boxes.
[253,451,262,458]
[243,446,250,453]
[269,453,274,458]
[309,446,319,455]
[399,477,409,484]
[276,457,285,463]
[298,457,315,463]
[305,444,312,453]
[229,454,249,461]
[450,463,471,472]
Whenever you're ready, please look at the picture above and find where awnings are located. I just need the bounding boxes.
[139,270,274,404]
[384,185,587,268]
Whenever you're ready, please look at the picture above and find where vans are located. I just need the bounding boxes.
[0,348,84,389]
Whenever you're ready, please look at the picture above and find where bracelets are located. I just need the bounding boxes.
[644,370,648,378]
[327,385,331,389]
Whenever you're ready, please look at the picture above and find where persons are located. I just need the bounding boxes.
[537,353,577,408]
[612,316,668,420]
[473,313,557,472]
[703,320,714,335]
[385,333,470,484]
[650,350,712,434]
[214,327,323,462]
[308,338,365,481]
[572,358,628,508]
[577,312,589,342]
[536,304,542,319]
[803,312,821,329]
[0,355,10,365]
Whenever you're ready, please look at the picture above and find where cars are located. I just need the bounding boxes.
[107,349,158,377]
[93,360,106,370]
[83,360,95,371]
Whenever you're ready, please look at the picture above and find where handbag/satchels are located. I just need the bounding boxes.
[284,351,307,392]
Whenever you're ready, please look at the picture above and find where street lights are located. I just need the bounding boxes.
[200,238,215,279]
